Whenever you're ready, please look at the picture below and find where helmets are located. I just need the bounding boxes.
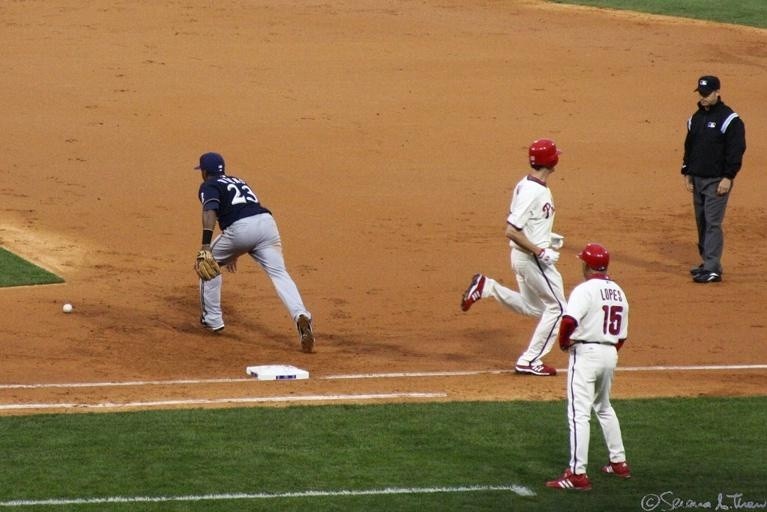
[529,139,563,168]
[194,152,224,175]
[576,244,609,270]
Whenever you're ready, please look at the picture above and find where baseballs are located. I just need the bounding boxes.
[62,303,73,312]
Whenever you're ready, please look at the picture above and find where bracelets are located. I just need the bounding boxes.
[201,228,214,247]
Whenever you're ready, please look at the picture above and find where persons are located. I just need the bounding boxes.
[460,139,568,377]
[545,242,630,491]
[681,75,747,283]
[192,151,315,354]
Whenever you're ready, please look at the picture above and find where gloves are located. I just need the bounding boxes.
[536,248,560,266]
[550,233,565,250]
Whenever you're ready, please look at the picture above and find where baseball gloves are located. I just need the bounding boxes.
[194,250,222,282]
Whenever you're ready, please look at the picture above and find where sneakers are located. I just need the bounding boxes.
[545,468,592,491]
[200,315,224,333]
[690,267,721,283]
[461,273,485,311]
[600,463,630,477]
[515,364,556,376]
[297,314,314,353]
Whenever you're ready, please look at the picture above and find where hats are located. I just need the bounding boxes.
[694,76,720,98]
